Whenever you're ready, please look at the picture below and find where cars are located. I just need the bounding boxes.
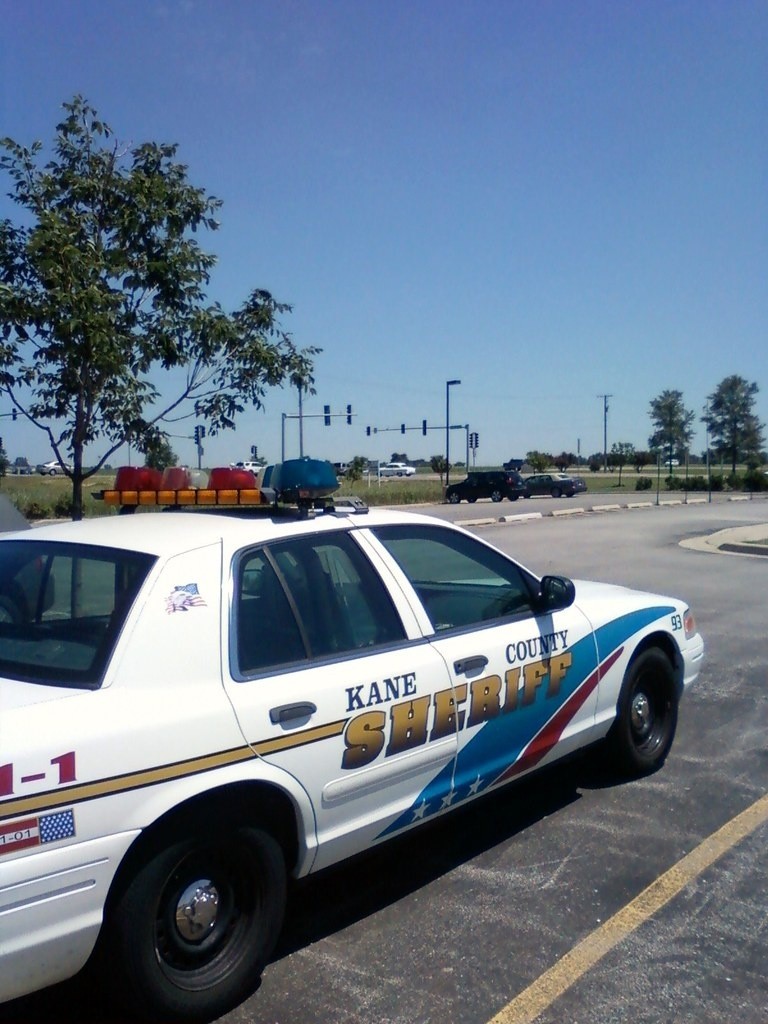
[0,457,704,1013]
[665,459,679,467]
[445,460,588,504]
[37,460,416,477]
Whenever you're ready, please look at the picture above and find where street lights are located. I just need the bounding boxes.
[469,432,479,448]
[194,425,257,458]
[445,380,461,486]
[347,405,351,425]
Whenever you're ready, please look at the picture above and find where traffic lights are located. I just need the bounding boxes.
[0,408,17,448]
